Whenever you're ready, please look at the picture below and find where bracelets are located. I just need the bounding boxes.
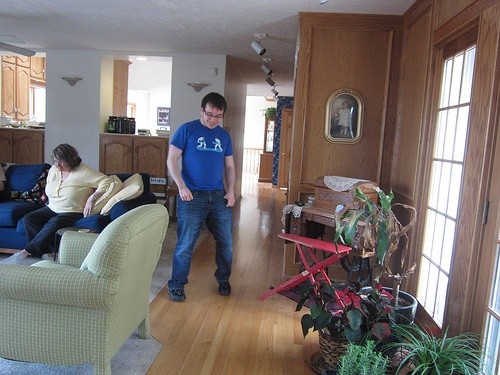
[92,194,97,200]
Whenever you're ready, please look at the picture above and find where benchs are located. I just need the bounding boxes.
[259,233,351,318]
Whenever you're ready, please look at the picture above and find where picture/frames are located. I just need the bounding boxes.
[156,107,170,126]
[324,88,365,144]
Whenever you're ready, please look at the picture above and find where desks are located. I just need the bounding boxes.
[281,204,364,285]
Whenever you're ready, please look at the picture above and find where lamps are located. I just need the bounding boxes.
[251,32,269,56]
[260,58,272,75]
[265,74,275,86]
[271,85,278,96]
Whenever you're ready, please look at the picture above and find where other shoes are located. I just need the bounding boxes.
[18,249,31,259]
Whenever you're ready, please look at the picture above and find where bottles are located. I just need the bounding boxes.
[107,115,136,134]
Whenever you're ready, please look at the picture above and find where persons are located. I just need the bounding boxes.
[14,144,110,258]
[167,92,235,302]
[336,101,351,136]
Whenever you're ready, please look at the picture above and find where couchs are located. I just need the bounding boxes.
[0,201,169,375]
[0,163,53,249]
[75,172,159,234]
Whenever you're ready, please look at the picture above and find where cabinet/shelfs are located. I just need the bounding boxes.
[99,133,169,200]
[1,56,46,120]
[0,128,44,165]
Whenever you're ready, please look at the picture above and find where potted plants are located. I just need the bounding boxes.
[294,183,500,375]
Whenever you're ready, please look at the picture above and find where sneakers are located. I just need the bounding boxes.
[219,281,231,295]
[168,288,186,301]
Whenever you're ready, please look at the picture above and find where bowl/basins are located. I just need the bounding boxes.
[157,130,170,136]
[10,120,22,128]
[0,116,12,126]
[138,129,150,136]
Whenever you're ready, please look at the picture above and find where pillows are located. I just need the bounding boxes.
[86,173,144,216]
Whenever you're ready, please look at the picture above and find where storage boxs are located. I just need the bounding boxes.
[314,175,379,215]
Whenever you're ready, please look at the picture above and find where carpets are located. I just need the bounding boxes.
[0,220,207,375]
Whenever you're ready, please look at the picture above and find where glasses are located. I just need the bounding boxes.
[204,109,224,118]
